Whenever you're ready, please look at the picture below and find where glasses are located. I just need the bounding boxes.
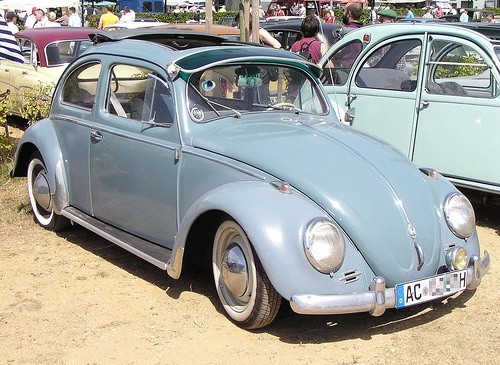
[380,19,390,23]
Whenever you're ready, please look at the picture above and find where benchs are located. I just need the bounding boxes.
[323,68,410,90]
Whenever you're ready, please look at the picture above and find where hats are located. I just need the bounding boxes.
[459,9,465,12]
[377,9,397,18]
[6,13,17,18]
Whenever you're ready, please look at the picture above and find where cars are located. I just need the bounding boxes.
[291,19,500,193]
[439,15,474,23]
[9,27,494,332]
[0,18,345,128]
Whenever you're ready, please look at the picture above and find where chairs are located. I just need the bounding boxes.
[439,82,465,96]
[402,79,417,91]
[130,94,174,124]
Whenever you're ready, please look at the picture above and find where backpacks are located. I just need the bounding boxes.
[290,41,316,79]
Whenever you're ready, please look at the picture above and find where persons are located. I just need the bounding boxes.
[235,1,500,104]
[6,4,136,55]
[196,10,202,24]
